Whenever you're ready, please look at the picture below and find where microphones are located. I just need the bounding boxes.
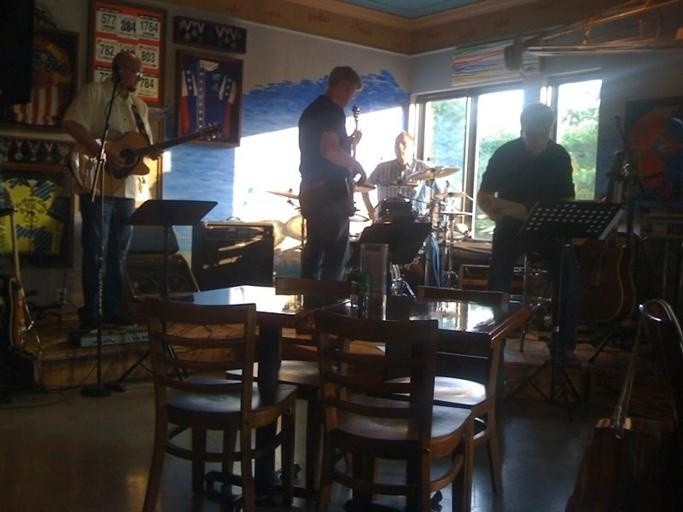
[352,105,361,127]
[112,62,125,86]
[613,145,646,196]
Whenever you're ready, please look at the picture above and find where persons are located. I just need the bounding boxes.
[361,132,442,286]
[64,50,154,324]
[298,66,366,281]
[478,104,579,298]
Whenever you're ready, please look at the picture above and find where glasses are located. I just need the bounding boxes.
[119,66,145,79]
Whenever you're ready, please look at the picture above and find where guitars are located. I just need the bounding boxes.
[577,149,636,322]
[71,122,225,199]
[2,189,38,352]
[342,105,362,219]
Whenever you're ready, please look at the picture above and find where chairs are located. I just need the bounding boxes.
[315,311,491,512]
[223,277,363,512]
[141,299,299,512]
[354,285,510,512]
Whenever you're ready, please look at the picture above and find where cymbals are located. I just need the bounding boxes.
[434,192,463,199]
[353,182,375,193]
[440,209,470,217]
[404,164,460,186]
[265,188,303,201]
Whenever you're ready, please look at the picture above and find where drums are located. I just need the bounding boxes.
[377,198,414,221]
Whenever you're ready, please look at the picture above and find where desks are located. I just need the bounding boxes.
[307,293,532,512]
[163,287,349,511]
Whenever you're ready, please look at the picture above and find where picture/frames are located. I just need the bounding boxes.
[624,97,683,206]
[0,30,79,173]
[175,50,244,149]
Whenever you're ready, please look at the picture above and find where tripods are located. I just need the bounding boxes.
[112,223,190,391]
[497,240,583,426]
[582,199,666,371]
[438,222,460,289]
